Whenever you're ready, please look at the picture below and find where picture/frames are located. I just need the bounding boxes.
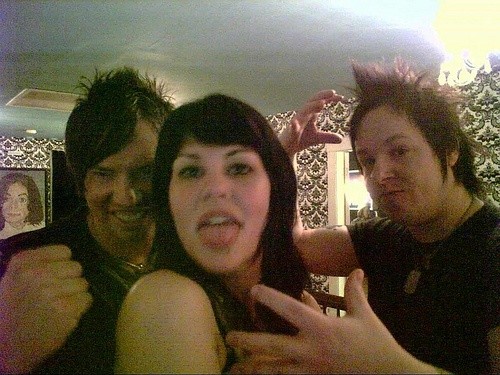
[0,168,48,241]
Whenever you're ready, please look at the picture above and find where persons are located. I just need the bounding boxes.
[114,91,325,375]
[0,64,195,375]
[225,55,500,375]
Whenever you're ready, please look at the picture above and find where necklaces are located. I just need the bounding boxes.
[403,194,474,294]
[119,252,158,274]
